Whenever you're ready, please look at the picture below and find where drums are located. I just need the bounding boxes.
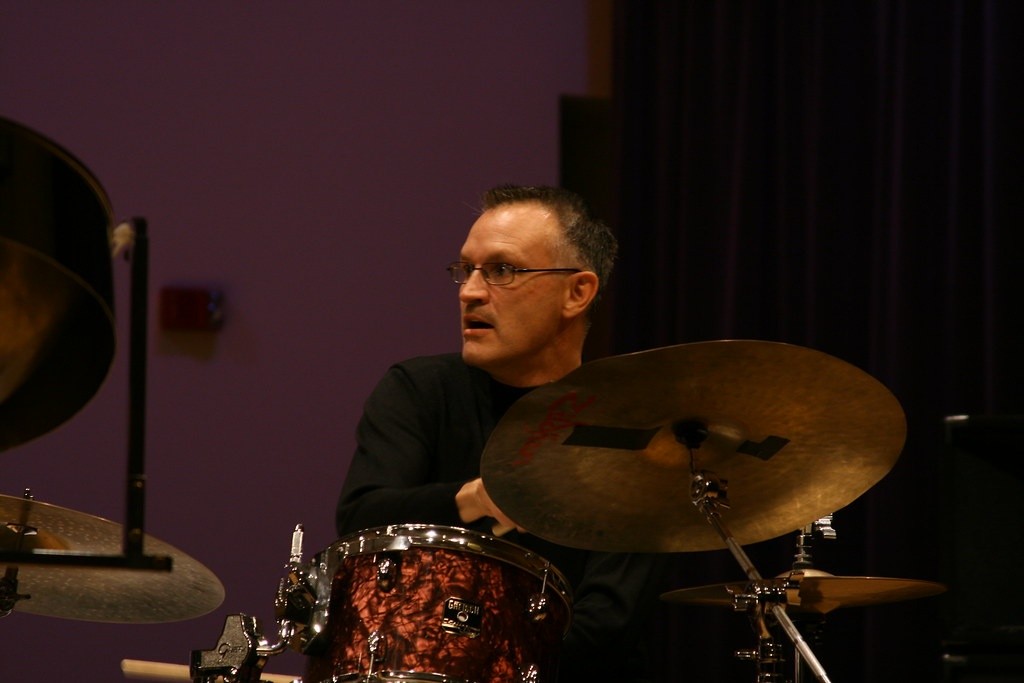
[1,115,119,456]
[301,522,575,683]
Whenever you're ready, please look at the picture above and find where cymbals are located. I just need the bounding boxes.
[0,492,227,626]
[660,565,949,613]
[479,335,914,557]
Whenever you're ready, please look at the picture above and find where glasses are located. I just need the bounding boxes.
[445,262,582,285]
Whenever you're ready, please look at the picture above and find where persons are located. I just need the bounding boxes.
[335,183,684,683]
